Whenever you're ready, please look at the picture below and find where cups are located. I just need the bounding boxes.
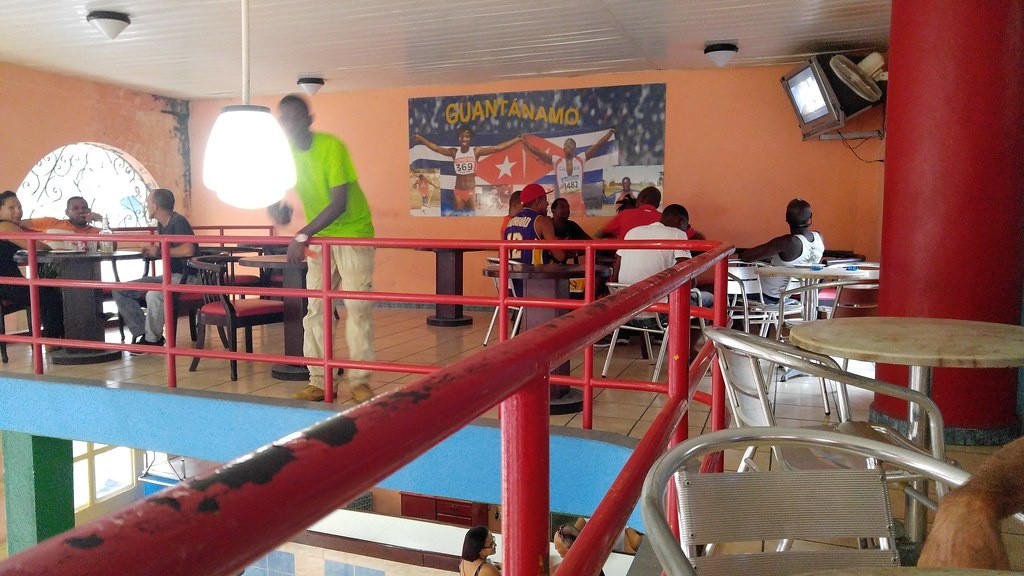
[86,239,98,253]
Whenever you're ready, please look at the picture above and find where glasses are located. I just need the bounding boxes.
[789,216,812,227]
[680,216,692,231]
[558,524,568,542]
[482,541,495,549]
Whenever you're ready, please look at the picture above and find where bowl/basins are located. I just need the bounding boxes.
[47,229,76,250]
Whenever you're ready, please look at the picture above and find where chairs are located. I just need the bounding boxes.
[483,248,1024,576]
[0,245,286,383]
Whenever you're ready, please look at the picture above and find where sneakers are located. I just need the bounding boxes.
[352,384,375,403]
[289,385,337,402]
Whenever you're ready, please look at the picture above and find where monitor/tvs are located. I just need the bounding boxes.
[780,53,873,136]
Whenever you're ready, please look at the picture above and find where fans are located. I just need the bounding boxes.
[829,52,889,103]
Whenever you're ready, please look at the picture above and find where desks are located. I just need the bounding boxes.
[14,250,144,365]
[789,316,1024,551]
[414,246,485,328]
[483,264,613,415]
[753,267,869,382]
[238,255,312,381]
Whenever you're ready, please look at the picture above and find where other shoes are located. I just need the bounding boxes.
[594,339,611,348]
[130,334,165,355]
[617,332,630,343]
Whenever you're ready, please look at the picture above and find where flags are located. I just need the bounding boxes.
[409,126,618,217]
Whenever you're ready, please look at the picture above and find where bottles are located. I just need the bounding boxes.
[98,213,114,252]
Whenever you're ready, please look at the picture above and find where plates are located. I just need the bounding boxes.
[48,248,85,254]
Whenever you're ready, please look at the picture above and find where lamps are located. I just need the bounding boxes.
[201,0,298,211]
[703,43,739,67]
[297,76,325,96]
[87,11,131,40]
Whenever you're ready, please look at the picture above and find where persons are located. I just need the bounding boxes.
[459,526,501,576]
[276,95,376,401]
[593,186,714,372]
[487,185,503,208]
[518,125,619,216]
[498,183,612,348]
[413,175,438,207]
[601,176,656,205]
[729,198,825,331]
[415,125,523,212]
[574,517,645,553]
[112,189,203,356]
[554,523,605,576]
[0,191,117,352]
[917,435,1024,571]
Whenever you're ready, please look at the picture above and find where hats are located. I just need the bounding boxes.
[520,184,555,204]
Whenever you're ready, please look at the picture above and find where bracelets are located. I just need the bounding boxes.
[294,233,308,242]
[635,530,644,536]
